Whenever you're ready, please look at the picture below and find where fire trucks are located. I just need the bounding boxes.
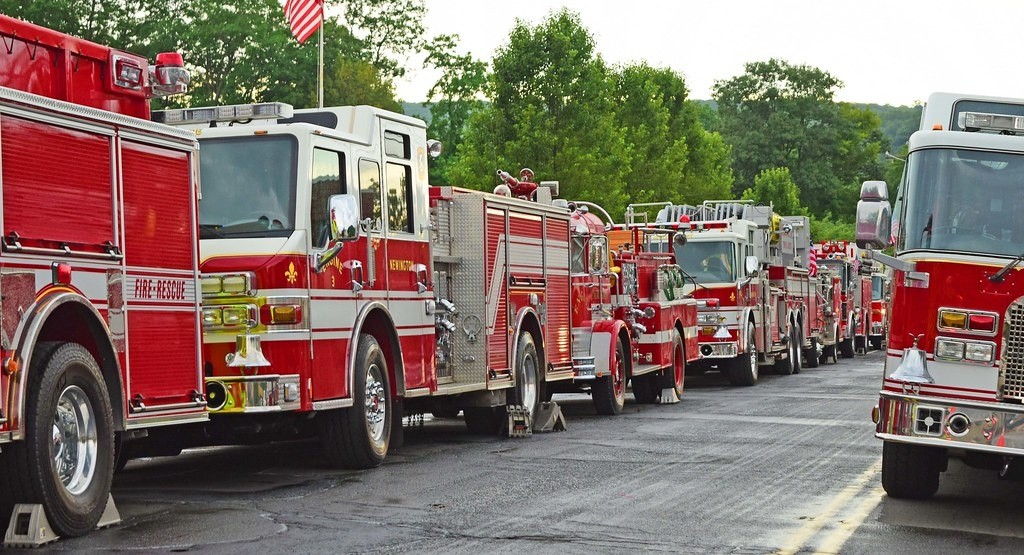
[0,16,1024,547]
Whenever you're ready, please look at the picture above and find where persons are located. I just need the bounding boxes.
[699,246,732,274]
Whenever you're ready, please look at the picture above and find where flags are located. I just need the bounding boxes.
[284,0,324,43]
